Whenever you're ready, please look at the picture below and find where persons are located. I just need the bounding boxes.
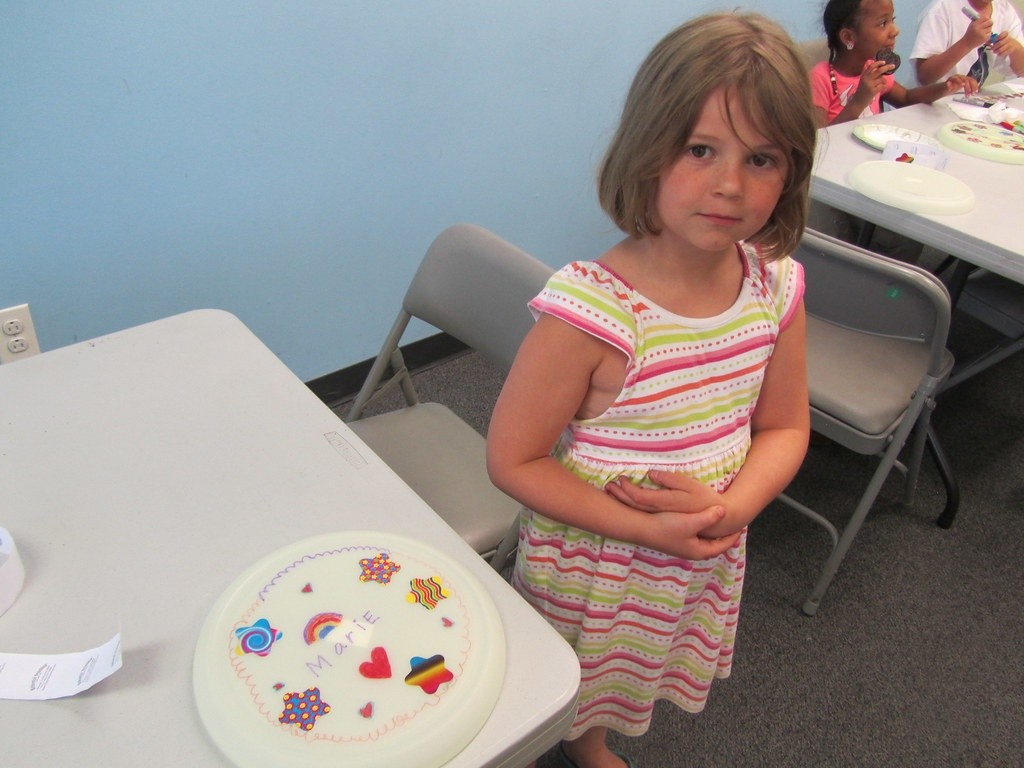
[486,0,1024,768]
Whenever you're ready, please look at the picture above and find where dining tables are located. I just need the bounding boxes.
[0,309,583,767]
[808,76,1024,386]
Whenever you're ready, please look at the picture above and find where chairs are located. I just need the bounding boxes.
[767,226,956,615]
[342,222,559,580]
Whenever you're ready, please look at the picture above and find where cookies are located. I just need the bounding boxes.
[876,48,901,75]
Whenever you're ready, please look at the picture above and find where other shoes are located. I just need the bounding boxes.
[559,740,637,768]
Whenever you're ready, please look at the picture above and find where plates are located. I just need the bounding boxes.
[938,121,1024,164]
[191,530,505,768]
[852,123,940,153]
[847,160,975,215]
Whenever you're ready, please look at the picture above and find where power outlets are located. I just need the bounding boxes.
[0,301,39,367]
